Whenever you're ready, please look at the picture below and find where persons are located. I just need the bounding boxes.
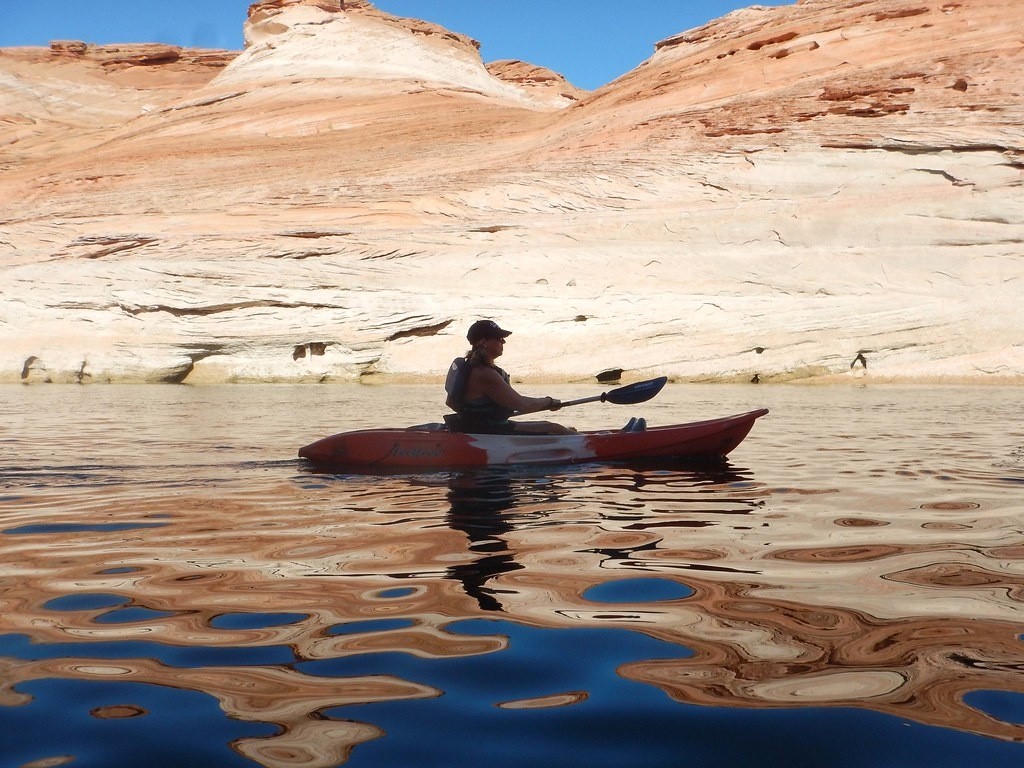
[459,320,647,434]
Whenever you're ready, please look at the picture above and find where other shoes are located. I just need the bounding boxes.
[623,417,636,432]
[632,418,647,431]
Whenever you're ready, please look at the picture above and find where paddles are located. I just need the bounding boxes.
[405,375,668,431]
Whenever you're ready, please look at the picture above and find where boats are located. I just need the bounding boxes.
[297,409,771,475]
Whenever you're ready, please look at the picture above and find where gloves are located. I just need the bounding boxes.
[547,396,562,411]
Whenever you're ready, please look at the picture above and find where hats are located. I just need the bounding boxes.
[468,319,512,344]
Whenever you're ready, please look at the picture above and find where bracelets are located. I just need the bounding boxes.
[545,396,553,408]
